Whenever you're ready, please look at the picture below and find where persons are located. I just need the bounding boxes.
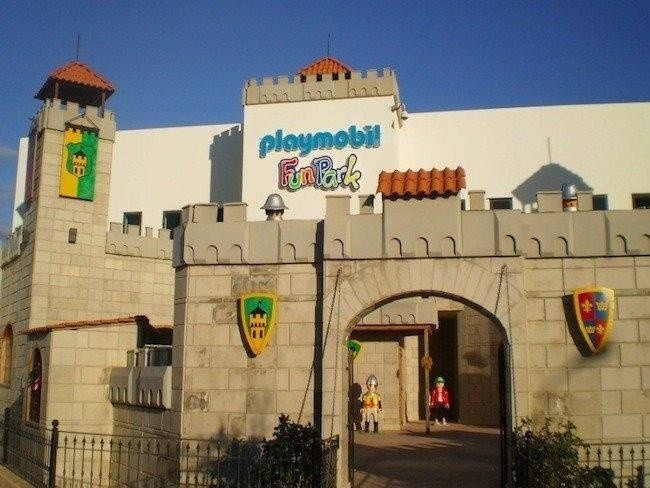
[360,374,382,432]
[430,375,450,425]
